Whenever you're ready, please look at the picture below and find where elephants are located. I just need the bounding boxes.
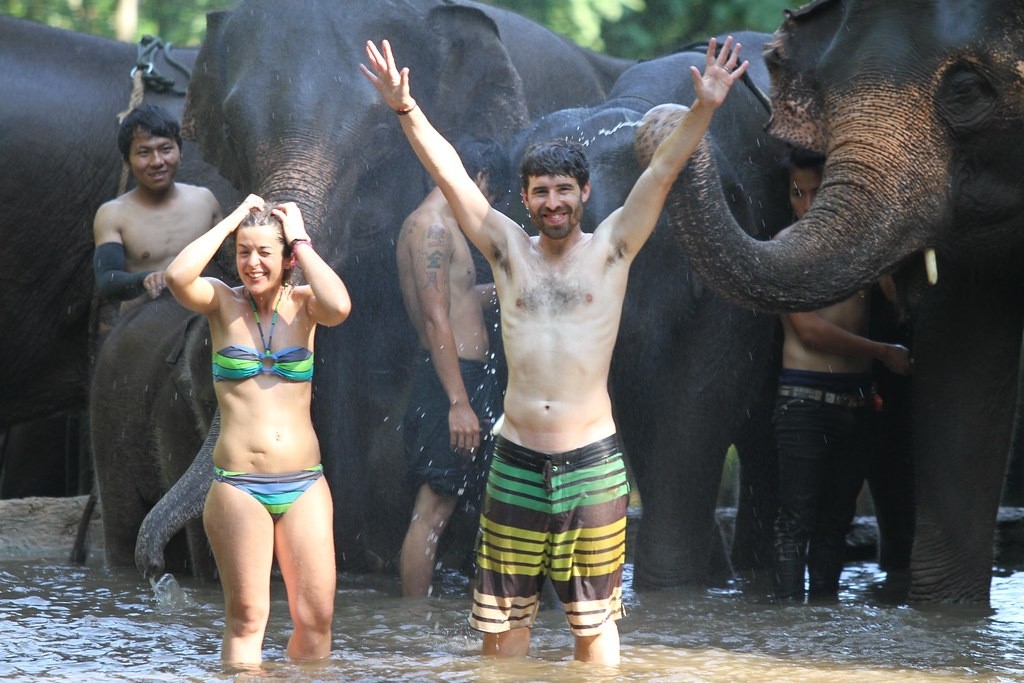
[0,0,1024,623]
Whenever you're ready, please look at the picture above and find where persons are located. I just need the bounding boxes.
[164,192,351,665]
[396,138,507,598]
[359,36,749,662]
[773,158,915,596]
[94,109,220,320]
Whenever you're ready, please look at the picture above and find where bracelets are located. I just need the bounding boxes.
[395,100,416,115]
[289,241,310,267]
[291,239,306,245]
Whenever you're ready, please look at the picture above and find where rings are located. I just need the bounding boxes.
[722,66,731,74]
